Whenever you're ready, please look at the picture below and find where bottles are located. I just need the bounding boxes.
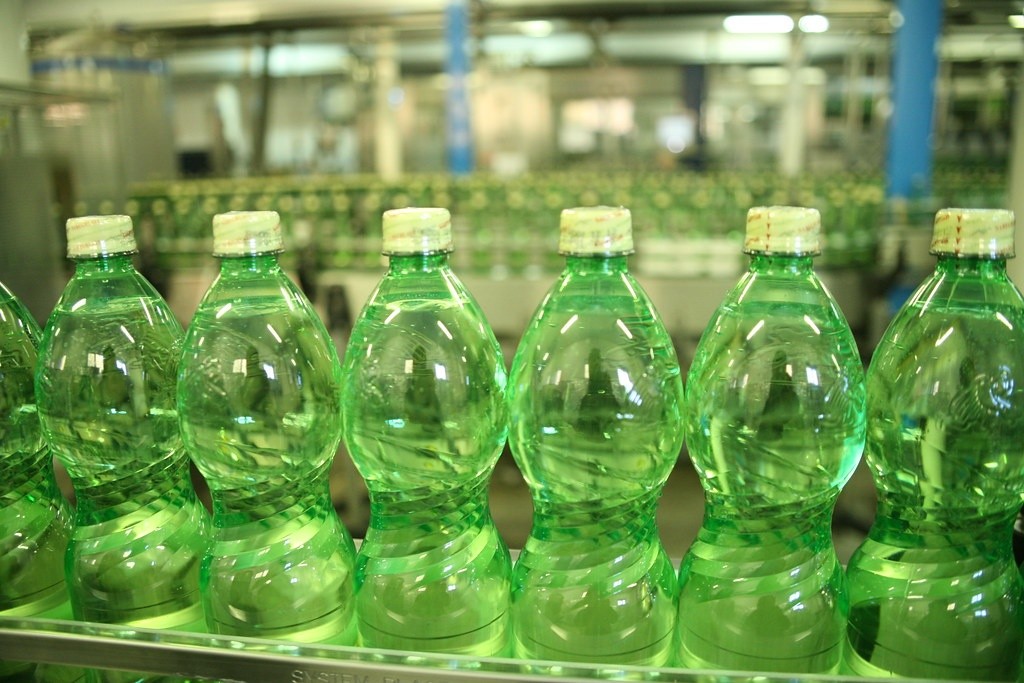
[0,152,1024,683]
